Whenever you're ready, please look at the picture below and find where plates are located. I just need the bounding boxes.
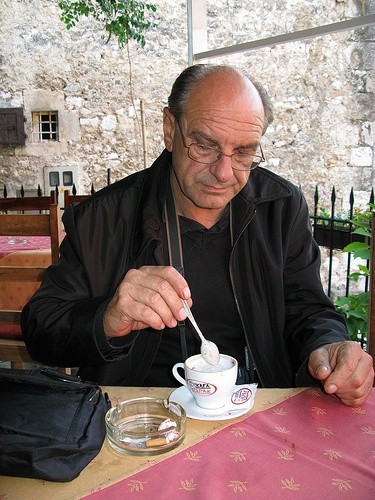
[168,385,254,422]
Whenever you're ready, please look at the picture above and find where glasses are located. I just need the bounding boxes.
[172,114,266,171]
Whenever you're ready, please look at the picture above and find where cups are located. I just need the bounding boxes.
[172,353,239,410]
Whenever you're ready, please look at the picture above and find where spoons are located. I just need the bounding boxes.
[182,300,220,367]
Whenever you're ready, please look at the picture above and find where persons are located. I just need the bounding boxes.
[21,64,375,407]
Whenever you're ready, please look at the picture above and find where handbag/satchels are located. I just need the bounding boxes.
[0,365,113,484]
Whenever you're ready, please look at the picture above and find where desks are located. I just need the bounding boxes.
[0,230,67,339]
[0,386,375,500]
